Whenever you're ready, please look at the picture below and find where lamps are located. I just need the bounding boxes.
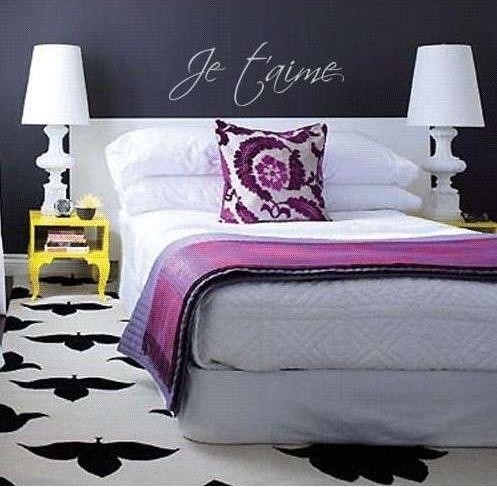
[21,44,90,216]
[406,44,485,218]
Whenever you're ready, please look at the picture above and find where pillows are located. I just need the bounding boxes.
[215,118,333,224]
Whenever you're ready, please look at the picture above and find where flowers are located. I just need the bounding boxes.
[74,194,99,208]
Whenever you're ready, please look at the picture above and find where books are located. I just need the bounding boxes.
[42,228,89,254]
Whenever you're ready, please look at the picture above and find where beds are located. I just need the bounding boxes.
[104,126,497,448]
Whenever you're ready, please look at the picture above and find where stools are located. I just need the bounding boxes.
[29,210,111,302]
[438,218,497,235]
